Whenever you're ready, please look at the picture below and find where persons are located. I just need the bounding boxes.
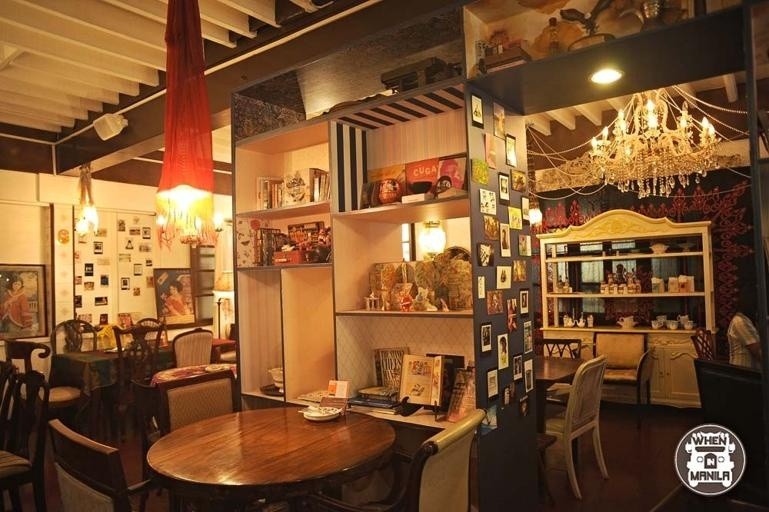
[726,289,764,372]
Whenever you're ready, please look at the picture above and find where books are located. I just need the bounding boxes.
[350,345,408,408]
[253,167,329,209]
[427,353,479,423]
[399,355,443,407]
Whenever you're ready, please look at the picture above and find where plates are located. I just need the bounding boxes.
[617,321,640,329]
[303,406,339,422]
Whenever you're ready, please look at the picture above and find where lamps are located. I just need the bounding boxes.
[526,84,758,201]
[589,41,627,87]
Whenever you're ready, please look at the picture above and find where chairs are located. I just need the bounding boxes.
[112,325,162,441]
[543,354,610,500]
[156,370,236,511]
[49,418,153,512]
[400,410,483,512]
[691,328,715,365]
[134,318,169,350]
[542,339,581,361]
[0,370,52,511]
[3,338,89,433]
[172,328,214,367]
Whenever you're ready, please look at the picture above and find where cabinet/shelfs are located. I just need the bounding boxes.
[231,4,541,497]
[535,207,716,376]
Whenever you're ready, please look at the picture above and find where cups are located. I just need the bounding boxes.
[684,321,694,330]
[667,320,673,329]
[670,320,679,329]
[651,320,663,329]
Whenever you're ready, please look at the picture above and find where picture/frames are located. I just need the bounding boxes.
[153,267,196,327]
[0,262,49,339]
